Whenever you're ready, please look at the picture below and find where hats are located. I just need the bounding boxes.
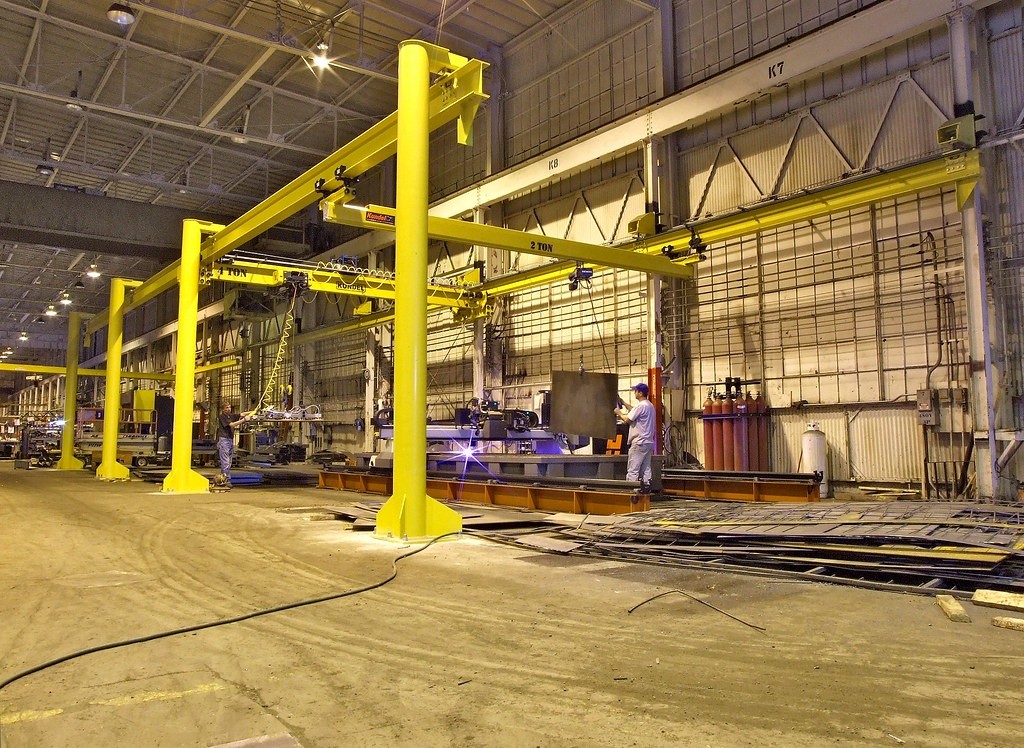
[630,383,649,394]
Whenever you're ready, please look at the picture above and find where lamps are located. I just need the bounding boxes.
[87,255,101,278]
[231,125,248,144]
[0,345,14,359]
[36,137,54,175]
[66,90,83,112]
[106,3,136,25]
[46,303,57,316]
[179,170,187,194]
[60,291,72,304]
[75,274,84,289]
[314,41,329,70]
[37,310,45,324]
[19,327,28,340]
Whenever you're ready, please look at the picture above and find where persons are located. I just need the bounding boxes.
[614,383,656,490]
[217,402,250,482]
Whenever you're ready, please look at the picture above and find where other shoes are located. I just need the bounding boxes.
[228,483,233,487]
